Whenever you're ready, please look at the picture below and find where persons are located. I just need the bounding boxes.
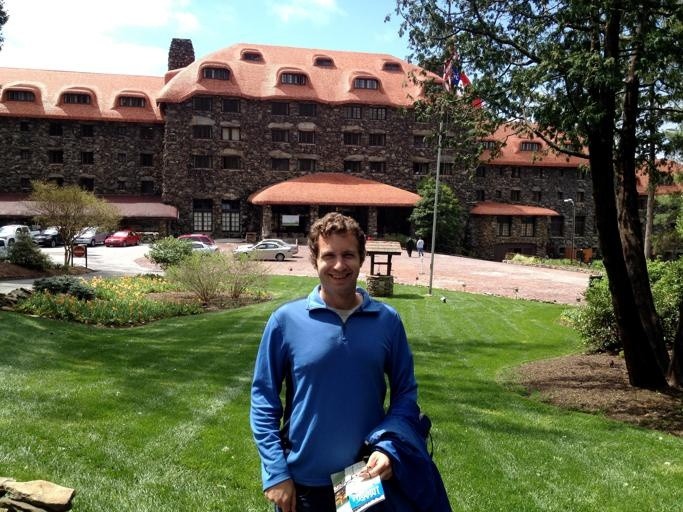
[415,234,426,258]
[404,236,414,257]
[244,208,452,512]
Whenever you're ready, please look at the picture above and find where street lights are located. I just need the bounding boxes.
[564,198,575,264]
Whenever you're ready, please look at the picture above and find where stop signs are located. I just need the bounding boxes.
[73,246,85,257]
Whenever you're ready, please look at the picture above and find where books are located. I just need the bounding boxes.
[330,460,385,512]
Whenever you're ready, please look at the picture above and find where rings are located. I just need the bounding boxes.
[368,472,371,479]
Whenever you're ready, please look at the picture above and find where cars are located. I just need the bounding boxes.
[32,230,64,248]
[71,227,111,246]
[0,225,40,247]
[105,231,141,246]
[177,234,218,254]
[233,239,299,261]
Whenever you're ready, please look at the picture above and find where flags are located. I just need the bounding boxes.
[440,37,482,112]
